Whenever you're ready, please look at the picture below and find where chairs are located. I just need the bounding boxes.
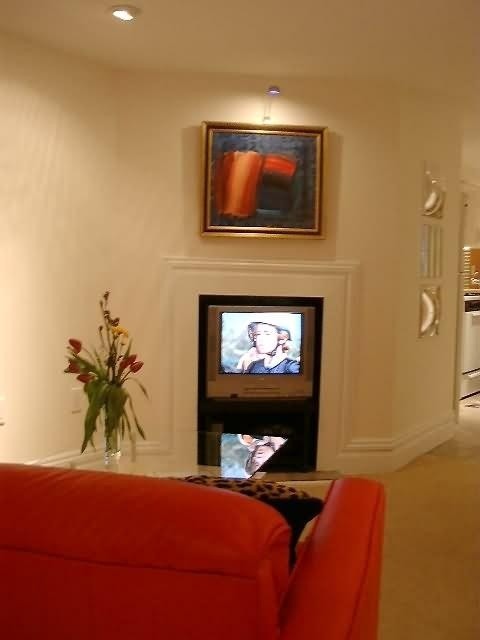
[0,461,384,640]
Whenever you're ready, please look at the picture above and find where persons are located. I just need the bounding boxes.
[235,325,264,373]
[244,312,300,374]
[232,433,288,475]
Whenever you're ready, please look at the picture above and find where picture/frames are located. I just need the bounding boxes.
[200,121,328,239]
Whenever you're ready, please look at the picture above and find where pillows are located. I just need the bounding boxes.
[166,476,323,574]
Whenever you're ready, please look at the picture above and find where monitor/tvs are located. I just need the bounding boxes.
[206,304,316,401]
[205,428,312,480]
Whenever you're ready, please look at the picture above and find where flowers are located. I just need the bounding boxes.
[65,291,152,455]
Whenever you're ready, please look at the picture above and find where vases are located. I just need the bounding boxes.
[104,398,122,460]
